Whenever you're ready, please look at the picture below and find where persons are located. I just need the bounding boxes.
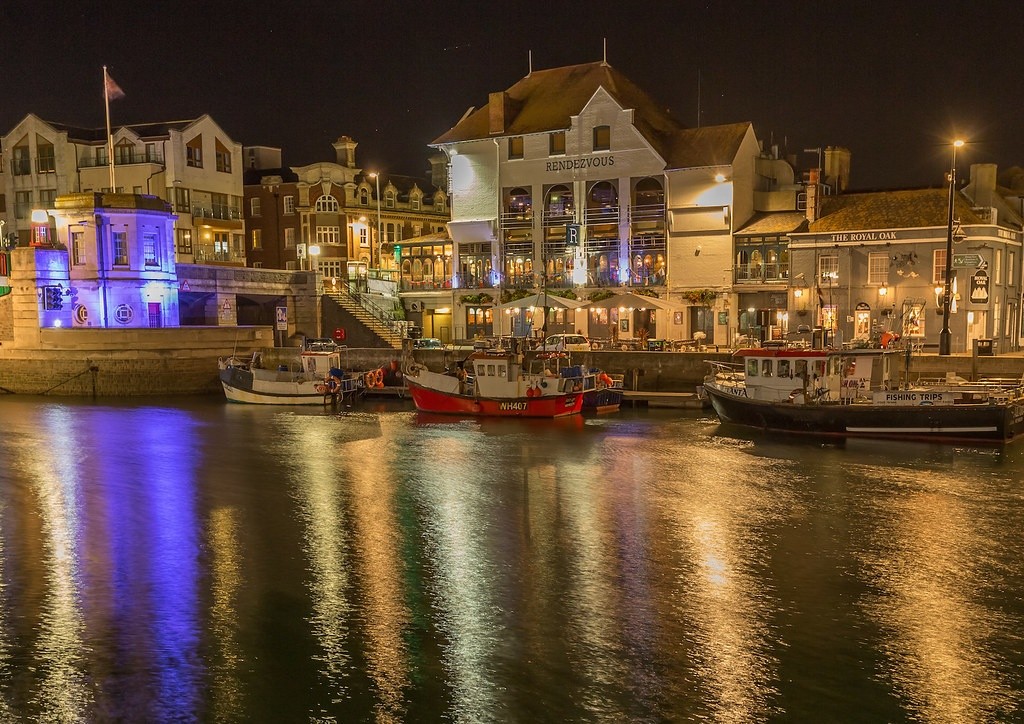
[456,365,468,396]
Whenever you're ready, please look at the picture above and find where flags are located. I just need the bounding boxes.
[105,72,124,99]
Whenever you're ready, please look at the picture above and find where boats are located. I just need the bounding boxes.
[401,336,598,418]
[701,275,1024,448]
[217,331,382,405]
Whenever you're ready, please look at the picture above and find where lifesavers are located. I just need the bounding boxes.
[553,352,566,357]
[325,376,341,394]
[536,352,556,359]
[366,372,375,388]
[375,369,384,385]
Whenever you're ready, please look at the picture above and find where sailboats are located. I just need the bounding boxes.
[524,219,625,415]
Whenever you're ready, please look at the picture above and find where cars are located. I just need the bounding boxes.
[535,333,591,352]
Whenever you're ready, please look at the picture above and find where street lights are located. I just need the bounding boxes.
[370,173,382,279]
[940,140,965,354]
